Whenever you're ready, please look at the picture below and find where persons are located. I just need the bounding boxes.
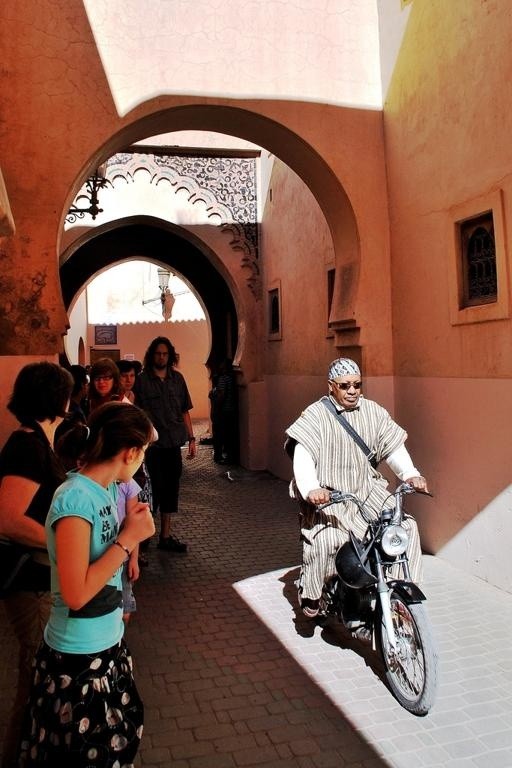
[112,474,145,629]
[5,399,162,768]
[114,357,144,406]
[0,359,78,765]
[279,356,430,642]
[134,334,198,552]
[89,356,160,568]
[54,363,93,446]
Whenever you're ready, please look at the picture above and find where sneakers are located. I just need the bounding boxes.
[298,588,319,617]
[159,535,186,552]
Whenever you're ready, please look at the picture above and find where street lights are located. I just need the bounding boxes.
[156,268,173,322]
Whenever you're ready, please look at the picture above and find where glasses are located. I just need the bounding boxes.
[154,352,169,357]
[332,380,361,390]
[95,375,112,380]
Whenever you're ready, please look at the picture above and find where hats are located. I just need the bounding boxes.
[116,360,142,376]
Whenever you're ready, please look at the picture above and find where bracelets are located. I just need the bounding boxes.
[185,436,197,444]
[109,537,133,557]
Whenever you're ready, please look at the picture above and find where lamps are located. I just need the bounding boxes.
[62,159,115,224]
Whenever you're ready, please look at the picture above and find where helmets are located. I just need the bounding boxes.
[336,530,379,590]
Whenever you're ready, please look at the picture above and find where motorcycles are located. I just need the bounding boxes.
[299,477,436,715]
